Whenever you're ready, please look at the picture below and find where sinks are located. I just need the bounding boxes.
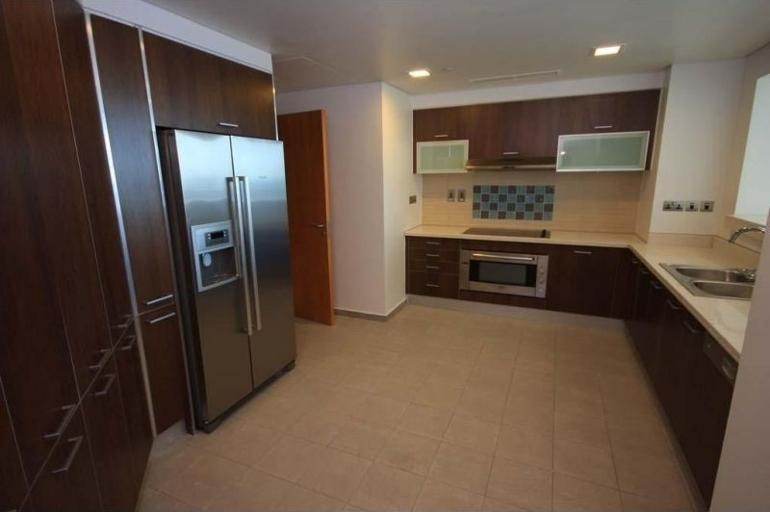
[660,263,756,279]
[687,280,753,298]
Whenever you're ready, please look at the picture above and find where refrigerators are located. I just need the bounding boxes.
[161,123,297,430]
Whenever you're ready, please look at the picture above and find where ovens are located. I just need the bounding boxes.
[459,250,548,299]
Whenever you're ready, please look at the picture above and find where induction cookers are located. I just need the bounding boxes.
[463,227,551,239]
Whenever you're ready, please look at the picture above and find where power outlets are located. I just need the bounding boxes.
[663,200,714,213]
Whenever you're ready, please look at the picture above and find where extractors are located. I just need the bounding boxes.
[466,157,556,171]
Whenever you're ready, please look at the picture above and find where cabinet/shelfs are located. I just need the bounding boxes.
[0,1,278,512]
[412,88,662,171]
[628,250,739,511]
[406,235,629,319]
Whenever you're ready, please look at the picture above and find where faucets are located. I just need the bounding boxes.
[728,226,764,245]
